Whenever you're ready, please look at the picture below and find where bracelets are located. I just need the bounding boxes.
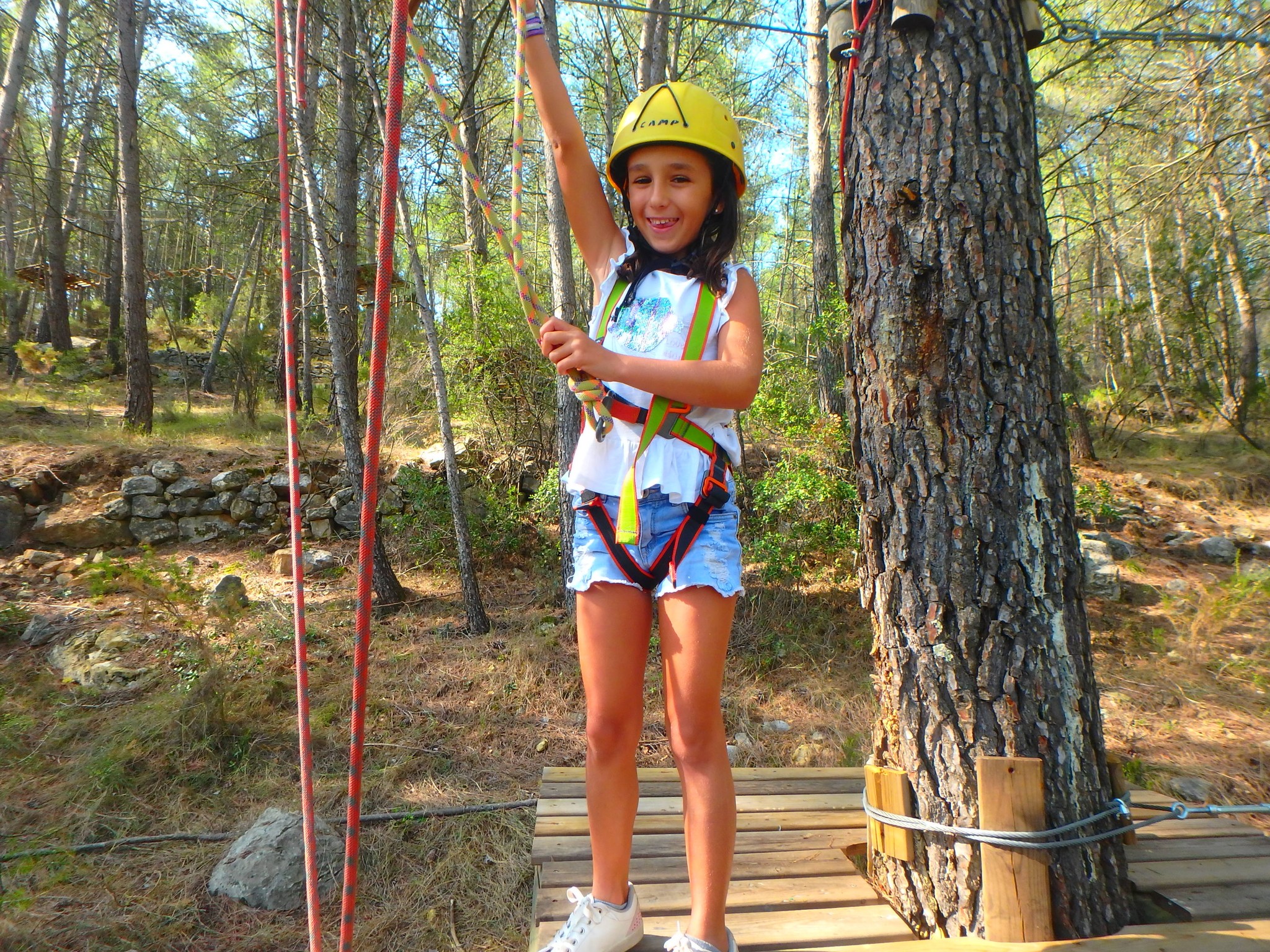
[512,9,545,43]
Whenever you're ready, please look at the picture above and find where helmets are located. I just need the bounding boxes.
[606,82,747,198]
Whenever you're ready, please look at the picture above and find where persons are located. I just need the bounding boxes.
[510,0,765,952]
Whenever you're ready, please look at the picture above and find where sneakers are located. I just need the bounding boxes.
[664,922,738,952]
[535,881,643,952]
